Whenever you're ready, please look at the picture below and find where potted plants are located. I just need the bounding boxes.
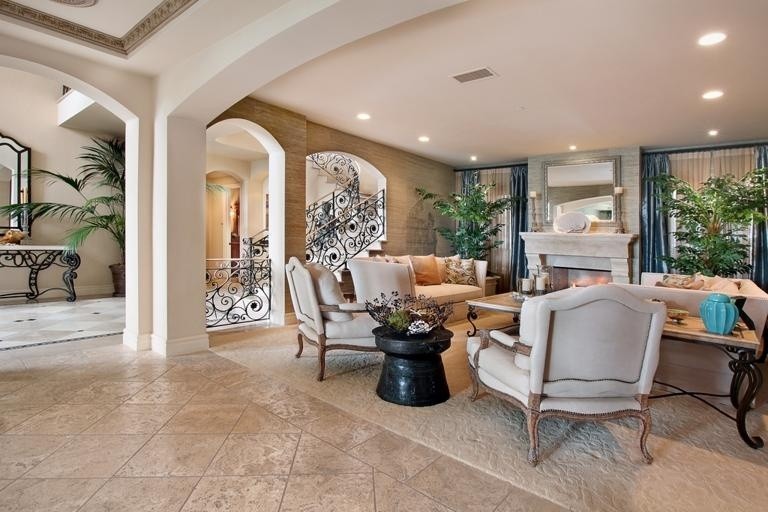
[0,131,228,299]
[411,174,531,295]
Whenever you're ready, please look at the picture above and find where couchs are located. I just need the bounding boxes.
[346,252,492,327]
[640,271,768,361]
[464,283,670,468]
[282,253,421,382]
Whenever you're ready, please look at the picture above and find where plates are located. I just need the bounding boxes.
[552,211,590,234]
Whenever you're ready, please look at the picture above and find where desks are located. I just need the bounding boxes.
[372,325,455,409]
[0,243,82,306]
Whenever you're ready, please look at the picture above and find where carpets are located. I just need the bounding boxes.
[206,291,768,512]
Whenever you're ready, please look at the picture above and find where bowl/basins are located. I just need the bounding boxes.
[0,231,29,243]
[666,308,689,323]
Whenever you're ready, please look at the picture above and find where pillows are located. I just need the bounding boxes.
[513,286,589,374]
[433,254,460,274]
[302,262,355,322]
[442,256,476,286]
[368,253,416,286]
[409,253,441,286]
[655,272,741,291]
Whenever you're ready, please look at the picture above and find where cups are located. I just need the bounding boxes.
[534,273,549,291]
[520,278,532,291]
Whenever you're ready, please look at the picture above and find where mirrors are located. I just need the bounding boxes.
[541,154,625,233]
[0,131,33,240]
